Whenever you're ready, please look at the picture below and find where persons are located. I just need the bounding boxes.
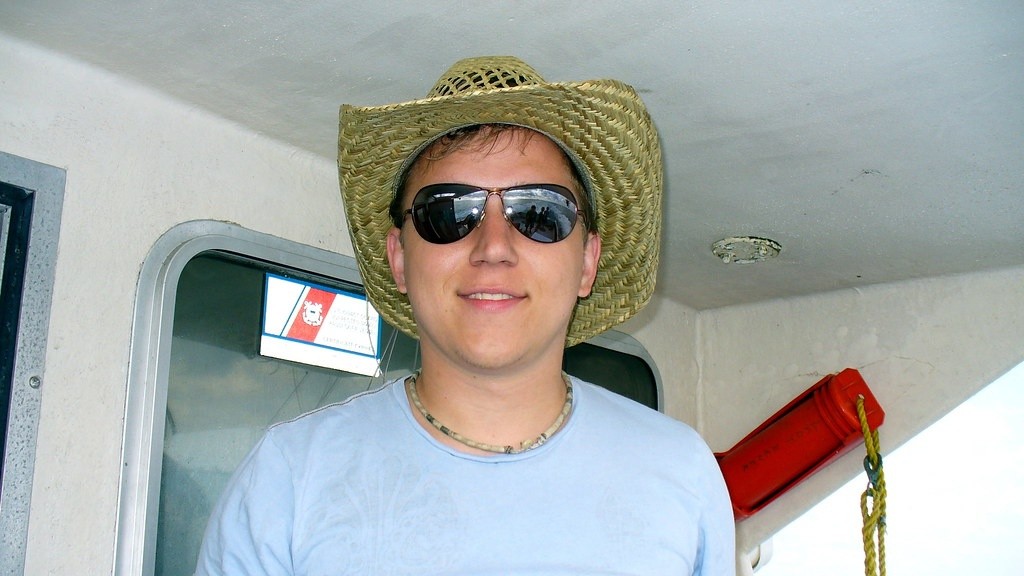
[195,55,736,576]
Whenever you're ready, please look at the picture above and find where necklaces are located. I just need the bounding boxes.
[408,366,573,454]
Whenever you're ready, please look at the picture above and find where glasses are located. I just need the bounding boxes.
[399,183,590,244]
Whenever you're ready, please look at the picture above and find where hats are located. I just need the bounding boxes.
[338,54,663,348]
